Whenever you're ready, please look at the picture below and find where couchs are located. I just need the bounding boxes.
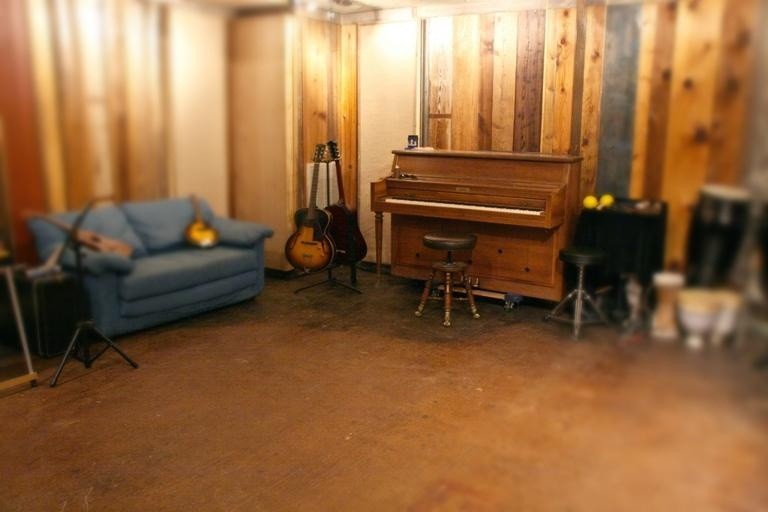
[24,197,274,338]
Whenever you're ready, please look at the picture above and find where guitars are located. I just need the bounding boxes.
[286,145,337,272]
[185,193,218,247]
[324,140,367,263]
[21,207,132,260]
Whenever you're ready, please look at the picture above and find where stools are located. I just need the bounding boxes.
[544,245,614,339]
[414,231,481,328]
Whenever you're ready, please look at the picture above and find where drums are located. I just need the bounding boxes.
[684,183,754,287]
[650,272,684,341]
[711,289,743,347]
[677,289,719,348]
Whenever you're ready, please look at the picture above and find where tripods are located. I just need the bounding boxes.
[45,245,140,386]
[293,163,365,295]
